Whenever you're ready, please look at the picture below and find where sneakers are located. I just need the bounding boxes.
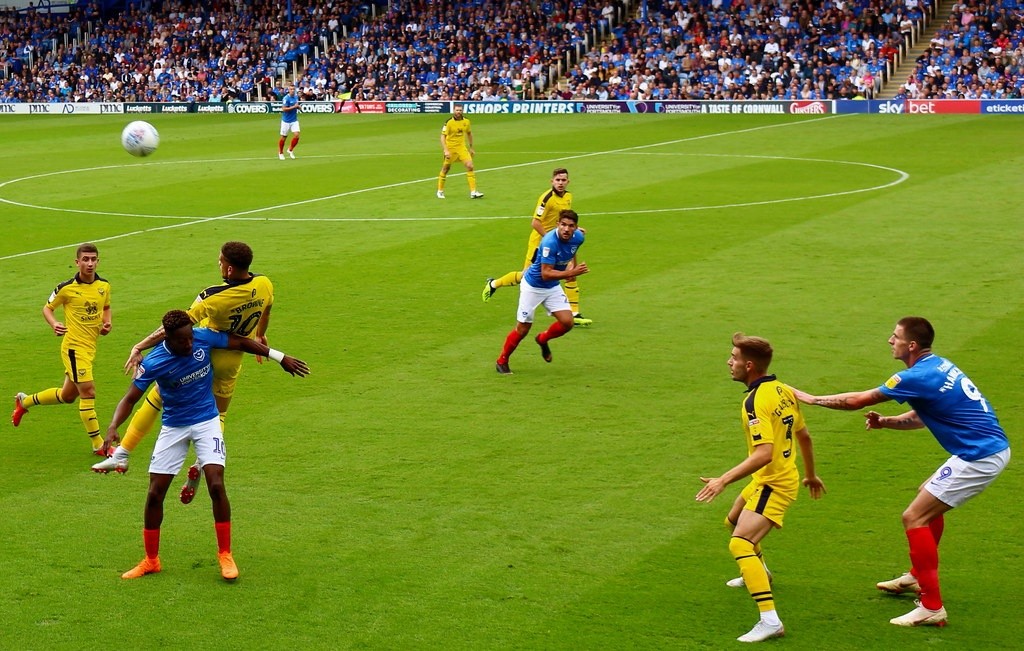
[726,570,772,587]
[93,445,117,458]
[180,464,202,504]
[122,554,161,578]
[279,152,285,160]
[481,278,497,303]
[876,571,922,594]
[287,149,295,159]
[12,392,28,426]
[496,360,513,375]
[470,192,483,198]
[91,453,128,475]
[437,191,445,198]
[536,334,553,362]
[736,620,785,642]
[218,551,238,579]
[573,314,592,327]
[889,602,947,626]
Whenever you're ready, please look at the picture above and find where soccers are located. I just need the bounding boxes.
[121,121,159,157]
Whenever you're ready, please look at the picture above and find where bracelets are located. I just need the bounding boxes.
[268,348,284,364]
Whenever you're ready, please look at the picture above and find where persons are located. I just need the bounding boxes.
[437,104,484,199]
[91,241,274,504]
[103,309,310,583]
[278,84,300,160]
[786,317,1010,628]
[497,209,589,377]
[695,334,826,642]
[0,0,1024,103]
[12,243,116,457]
[483,167,593,326]
